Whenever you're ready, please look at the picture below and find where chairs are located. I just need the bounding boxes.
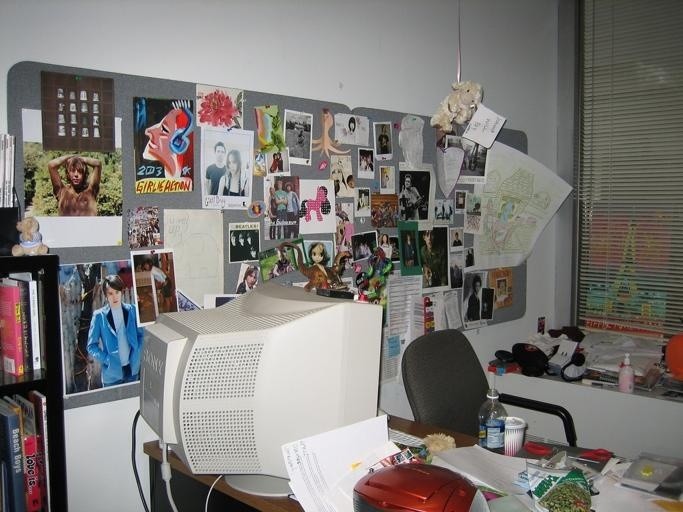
[401,329,576,446]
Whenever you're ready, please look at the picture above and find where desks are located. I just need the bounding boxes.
[141,415,683,510]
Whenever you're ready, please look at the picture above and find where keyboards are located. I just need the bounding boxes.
[387,426,423,446]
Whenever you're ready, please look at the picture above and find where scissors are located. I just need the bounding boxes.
[524,441,611,465]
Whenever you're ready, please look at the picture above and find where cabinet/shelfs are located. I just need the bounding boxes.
[0,254,69,511]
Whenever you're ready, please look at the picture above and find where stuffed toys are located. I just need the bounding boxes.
[428,79,483,134]
[10,216,49,256]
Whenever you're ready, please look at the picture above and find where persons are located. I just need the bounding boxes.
[345,116,359,145]
[404,233,415,266]
[142,101,194,178]
[125,206,162,249]
[236,265,259,293]
[229,231,259,261]
[218,149,250,196]
[86,274,148,387]
[398,173,426,221]
[267,179,299,221]
[308,241,330,267]
[420,229,448,287]
[269,152,286,173]
[357,192,368,213]
[204,142,228,195]
[378,124,390,154]
[360,152,373,172]
[466,275,482,321]
[141,258,176,313]
[452,232,463,246]
[47,153,102,217]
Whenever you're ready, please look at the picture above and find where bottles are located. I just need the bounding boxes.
[479,389,505,455]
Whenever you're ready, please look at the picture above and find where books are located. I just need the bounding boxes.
[0,130,17,210]
[579,331,665,392]
[0,389,55,512]
[621,449,683,494]
[432,443,552,496]
[0,271,48,385]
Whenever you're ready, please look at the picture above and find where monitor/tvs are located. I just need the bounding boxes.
[140,283,384,496]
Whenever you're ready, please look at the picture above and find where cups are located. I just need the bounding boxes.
[496,416,525,457]
